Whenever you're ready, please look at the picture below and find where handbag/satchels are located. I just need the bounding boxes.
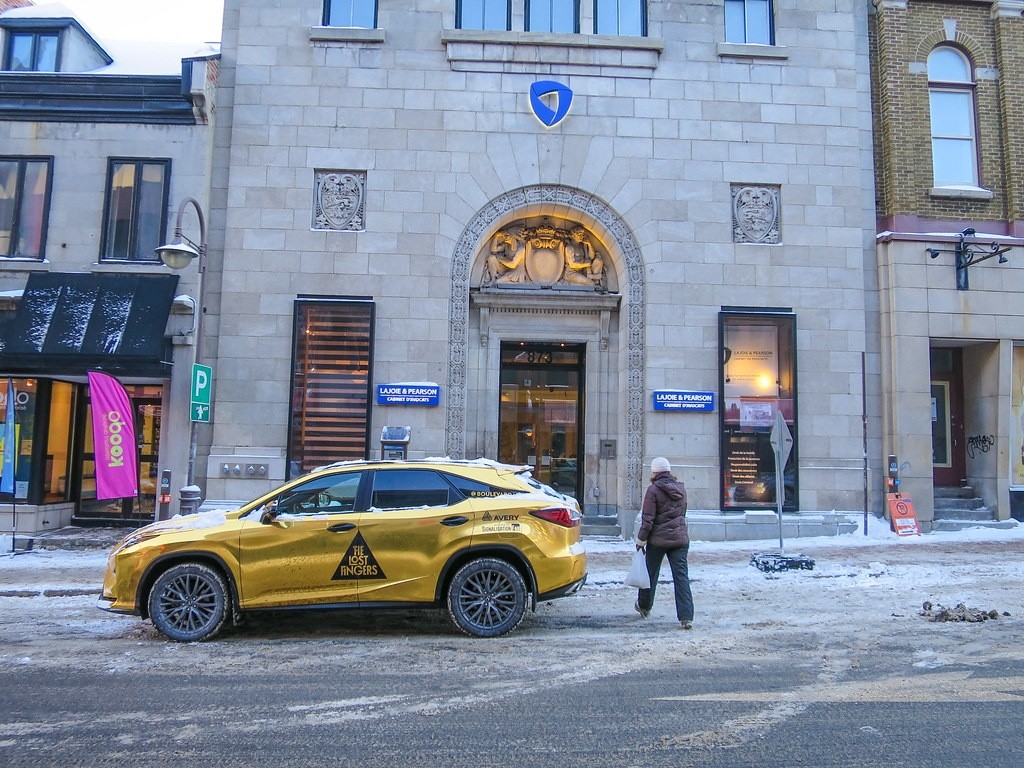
[624,547,651,589]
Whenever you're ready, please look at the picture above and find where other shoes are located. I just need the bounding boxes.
[681,620,692,629]
[635,601,649,618]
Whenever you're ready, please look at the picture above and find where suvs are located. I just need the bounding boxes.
[96,460,588,641]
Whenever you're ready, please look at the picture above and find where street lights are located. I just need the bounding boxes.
[154,196,209,515]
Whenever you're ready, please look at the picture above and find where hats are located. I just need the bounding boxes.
[650,457,670,472]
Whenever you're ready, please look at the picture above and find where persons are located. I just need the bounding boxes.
[725,403,740,420]
[633,458,695,629]
